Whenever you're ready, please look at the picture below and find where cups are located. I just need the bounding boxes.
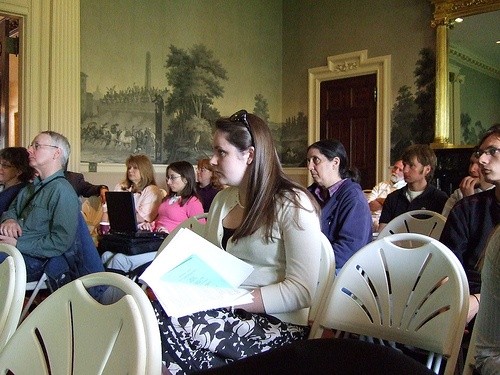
[99,222,111,235]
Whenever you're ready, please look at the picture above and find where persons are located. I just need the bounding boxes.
[306,139,374,338]
[195,158,224,220]
[103,153,207,305]
[152,111,322,375]
[404,122,500,375]
[0,131,81,283]
[366,144,448,323]
[63,158,108,205]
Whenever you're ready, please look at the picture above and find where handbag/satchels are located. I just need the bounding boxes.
[97,234,165,256]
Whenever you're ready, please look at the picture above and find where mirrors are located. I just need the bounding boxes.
[430,0,500,150]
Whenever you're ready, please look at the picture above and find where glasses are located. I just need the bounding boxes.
[166,175,183,179]
[229,109,255,146]
[27,143,58,150]
[0,161,15,169]
[475,148,500,158]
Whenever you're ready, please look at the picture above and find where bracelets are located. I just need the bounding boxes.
[230,306,234,314]
[472,295,479,303]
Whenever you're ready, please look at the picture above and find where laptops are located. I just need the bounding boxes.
[105,191,165,237]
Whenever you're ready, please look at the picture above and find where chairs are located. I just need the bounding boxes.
[312,232,470,375]
[17,211,110,325]
[308,233,336,338]
[0,242,27,352]
[141,212,209,291]
[337,210,447,338]
[0,271,163,375]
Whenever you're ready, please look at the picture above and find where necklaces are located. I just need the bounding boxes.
[239,202,244,209]
